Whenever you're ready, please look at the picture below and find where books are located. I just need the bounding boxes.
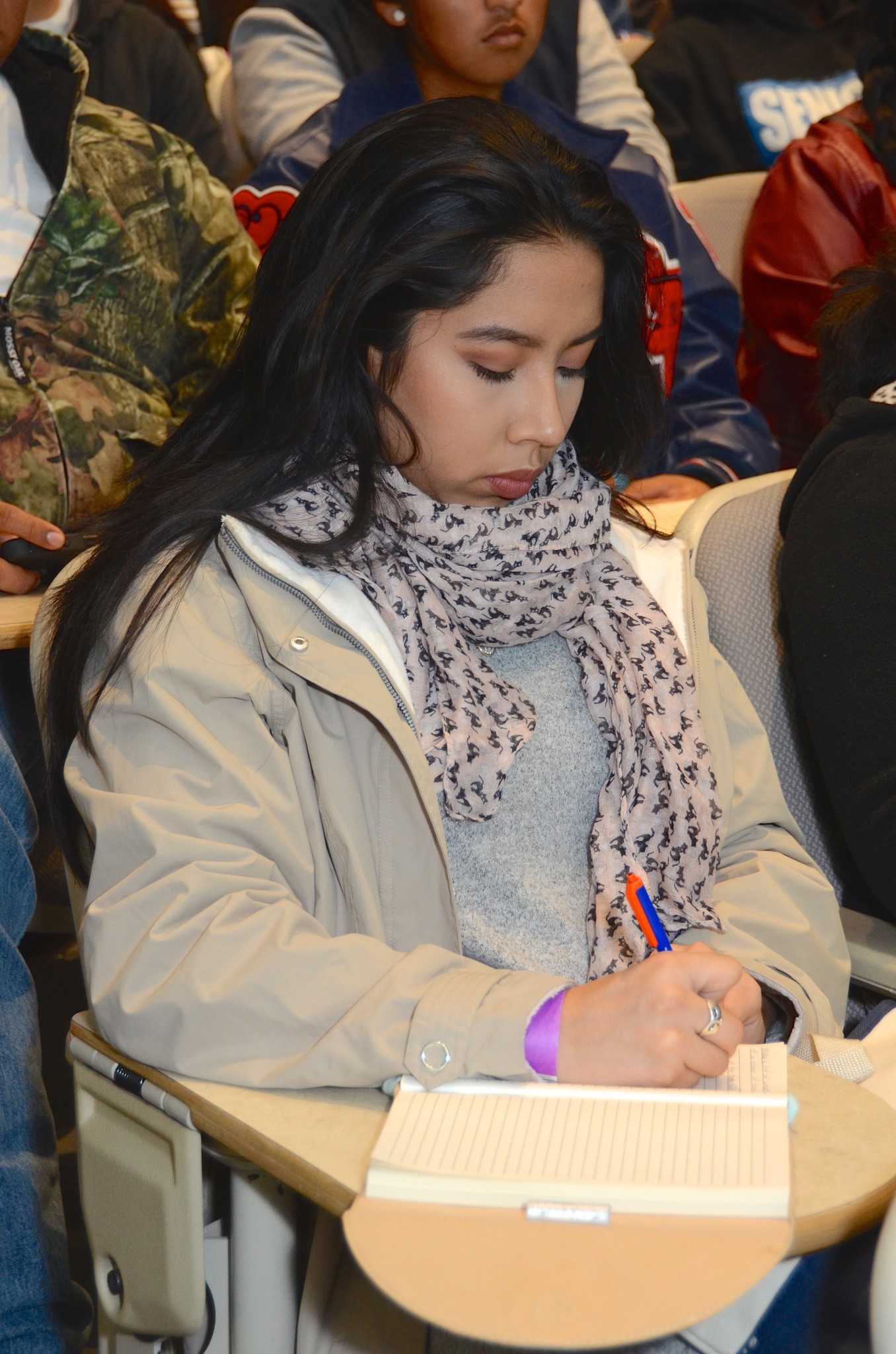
[365,1041,790,1218]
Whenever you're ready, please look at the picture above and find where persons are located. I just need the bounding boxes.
[0,0,896,1354]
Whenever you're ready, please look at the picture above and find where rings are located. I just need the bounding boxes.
[695,997,722,1038]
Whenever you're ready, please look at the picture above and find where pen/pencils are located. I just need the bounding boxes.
[620,867,669,954]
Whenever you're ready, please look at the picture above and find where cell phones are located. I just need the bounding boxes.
[0,531,101,584]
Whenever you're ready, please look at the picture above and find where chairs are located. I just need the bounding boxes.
[33,473,896,1354]
